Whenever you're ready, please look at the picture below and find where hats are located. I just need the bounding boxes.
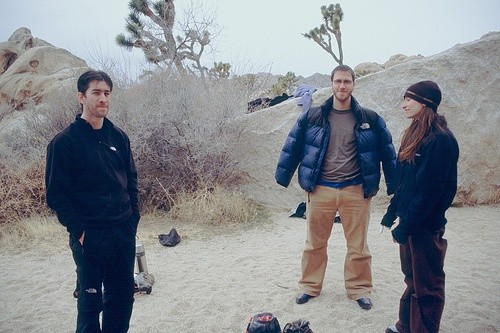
[404,81,441,112]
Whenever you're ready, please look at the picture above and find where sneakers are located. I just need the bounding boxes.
[357,299,372,309]
[296,293,316,304]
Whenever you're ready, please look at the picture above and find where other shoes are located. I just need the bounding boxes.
[385,326,399,333]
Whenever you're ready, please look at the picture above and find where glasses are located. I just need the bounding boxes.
[333,79,352,85]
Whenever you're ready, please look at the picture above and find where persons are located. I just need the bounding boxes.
[46,69,141,333]
[379,80,459,333]
[274,65,397,309]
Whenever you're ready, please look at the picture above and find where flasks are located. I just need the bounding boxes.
[135,244,148,275]
